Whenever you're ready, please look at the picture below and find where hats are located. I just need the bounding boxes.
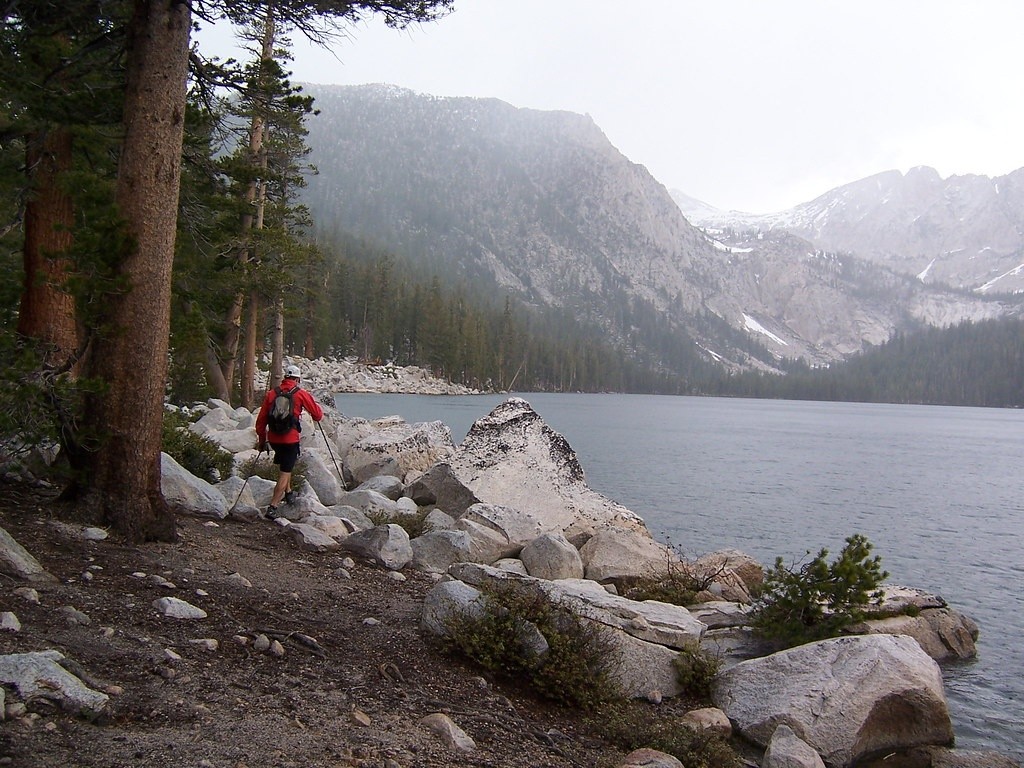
[285,365,301,377]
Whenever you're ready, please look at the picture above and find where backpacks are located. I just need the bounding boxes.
[269,385,301,434]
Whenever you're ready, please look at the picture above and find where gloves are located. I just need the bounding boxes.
[259,444,269,455]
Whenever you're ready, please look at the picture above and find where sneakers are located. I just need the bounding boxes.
[285,492,296,504]
[265,506,278,519]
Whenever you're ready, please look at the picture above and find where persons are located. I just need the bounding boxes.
[256,365,322,520]
